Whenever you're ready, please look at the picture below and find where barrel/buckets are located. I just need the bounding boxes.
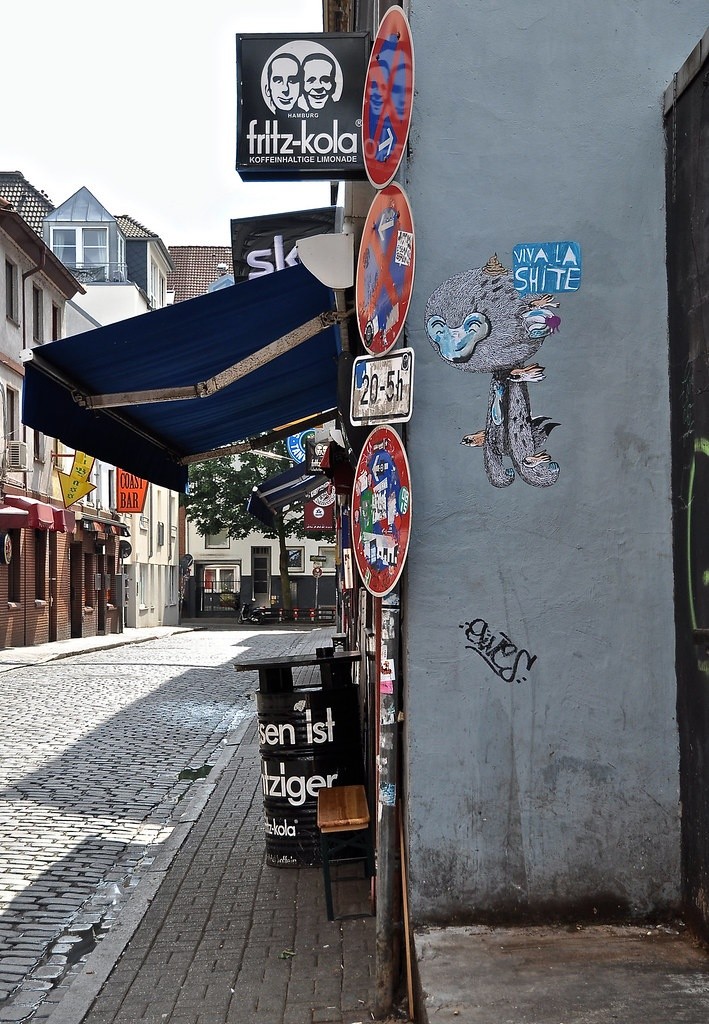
[255,685,366,869]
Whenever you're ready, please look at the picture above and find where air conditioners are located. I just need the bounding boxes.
[7,441,28,469]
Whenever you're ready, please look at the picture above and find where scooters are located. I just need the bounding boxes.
[237,602,265,626]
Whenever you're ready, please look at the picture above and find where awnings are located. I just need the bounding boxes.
[0,495,77,534]
[246,461,329,529]
[80,512,131,537]
[21,264,341,495]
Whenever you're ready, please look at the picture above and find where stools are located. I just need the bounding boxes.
[317,785,373,920]
[331,632,346,652]
